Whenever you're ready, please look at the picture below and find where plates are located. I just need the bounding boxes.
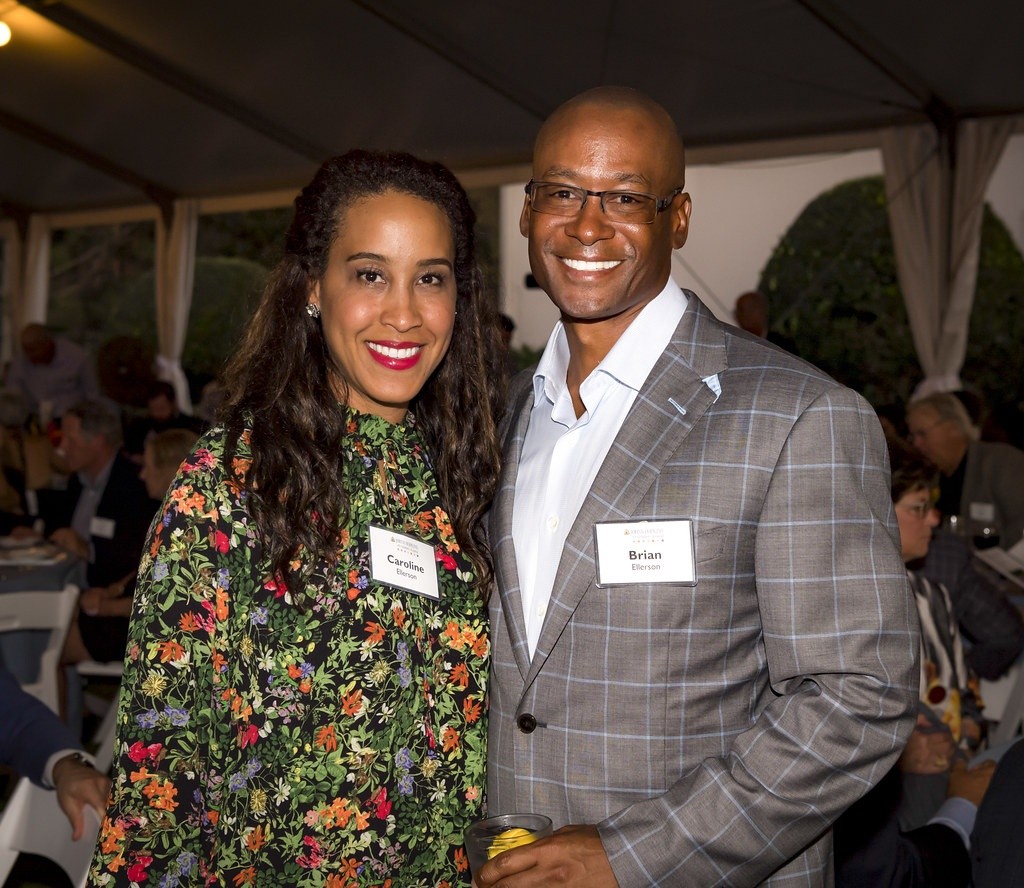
[0,551,67,565]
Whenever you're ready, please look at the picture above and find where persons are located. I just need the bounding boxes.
[840,392,1024,888]
[2,322,210,888]
[84,86,924,888]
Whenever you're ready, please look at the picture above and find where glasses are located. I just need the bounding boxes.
[524,176,684,224]
[896,501,931,521]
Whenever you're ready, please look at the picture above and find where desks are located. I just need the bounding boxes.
[0,528,90,717]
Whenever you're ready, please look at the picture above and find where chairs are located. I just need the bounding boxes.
[0,583,79,716]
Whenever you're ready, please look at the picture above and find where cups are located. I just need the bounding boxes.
[462,813,553,882]
